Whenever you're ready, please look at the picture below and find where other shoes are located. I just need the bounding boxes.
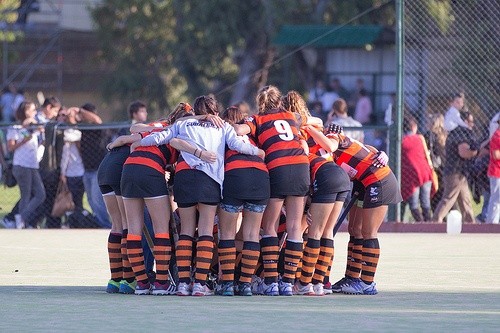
[14,213,26,228]
[3,215,15,229]
[324,282,333,294]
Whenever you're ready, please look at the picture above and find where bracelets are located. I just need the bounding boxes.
[108,143,112,150]
[431,167,434,170]
[193,149,202,157]
[78,108,82,113]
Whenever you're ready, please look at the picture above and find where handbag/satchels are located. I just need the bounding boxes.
[51,179,75,217]
[6,163,18,187]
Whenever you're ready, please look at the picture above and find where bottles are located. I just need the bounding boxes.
[446,210,462,234]
[21,131,32,139]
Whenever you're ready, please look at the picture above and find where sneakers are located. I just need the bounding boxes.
[191,282,215,296]
[134,280,149,295]
[279,280,294,296]
[176,281,191,296]
[150,281,176,295]
[119,280,137,294]
[256,280,279,296]
[332,276,365,295]
[235,282,252,296]
[292,281,315,296]
[359,278,378,295]
[313,283,325,296]
[106,279,119,294]
[221,281,234,296]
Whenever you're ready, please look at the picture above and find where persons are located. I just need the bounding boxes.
[0,86,500,295]
